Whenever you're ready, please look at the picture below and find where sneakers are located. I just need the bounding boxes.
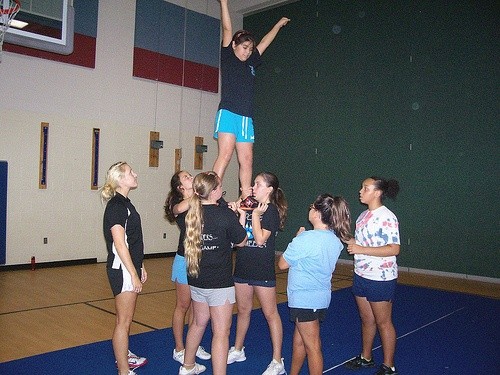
[173,349,185,365]
[196,346,212,360]
[262,357,287,375]
[115,349,147,369]
[346,353,377,369]
[128,369,138,375]
[178,362,207,375]
[227,346,246,364]
[240,196,259,208]
[217,191,229,208]
[373,362,397,375]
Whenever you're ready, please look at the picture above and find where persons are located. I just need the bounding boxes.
[212,0,290,208]
[279,194,355,375]
[163,171,287,375]
[97,162,147,375]
[341,176,401,375]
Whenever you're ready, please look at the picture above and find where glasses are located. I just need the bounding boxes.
[309,204,317,211]
